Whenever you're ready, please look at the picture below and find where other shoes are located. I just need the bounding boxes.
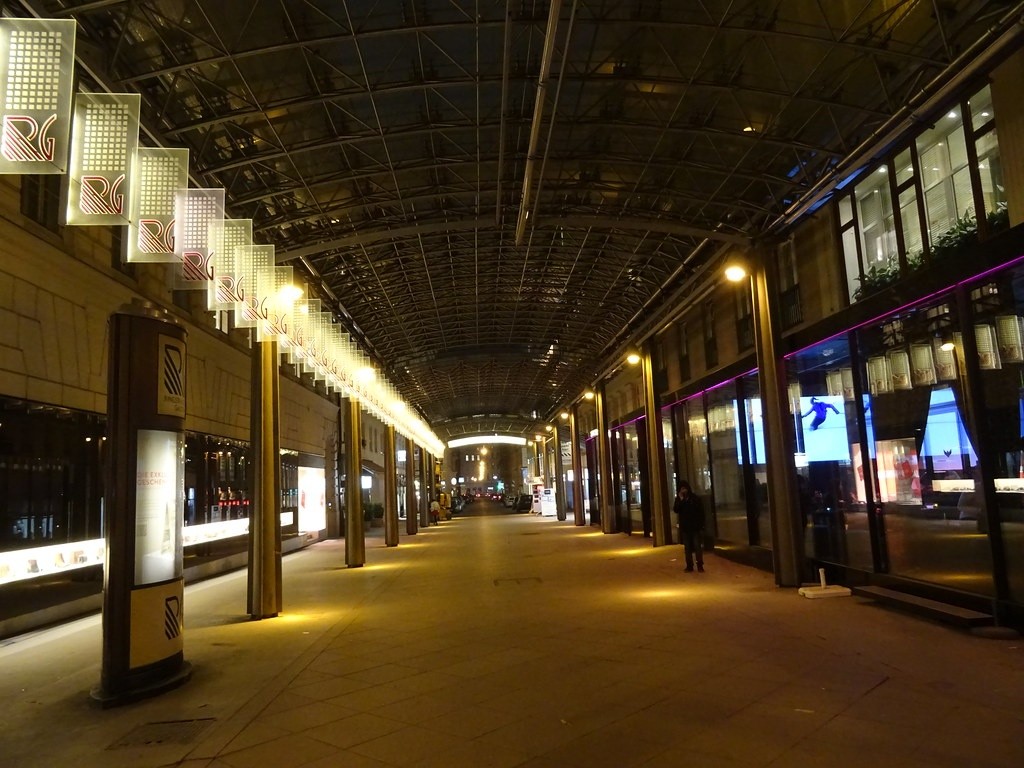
[698,566,704,573]
[684,567,694,573]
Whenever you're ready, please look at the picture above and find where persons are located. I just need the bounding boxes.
[429,499,440,526]
[801,397,840,431]
[672,482,706,572]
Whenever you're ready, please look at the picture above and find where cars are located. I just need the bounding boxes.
[464,492,504,504]
[451,496,465,512]
[505,495,516,507]
[515,494,533,513]
